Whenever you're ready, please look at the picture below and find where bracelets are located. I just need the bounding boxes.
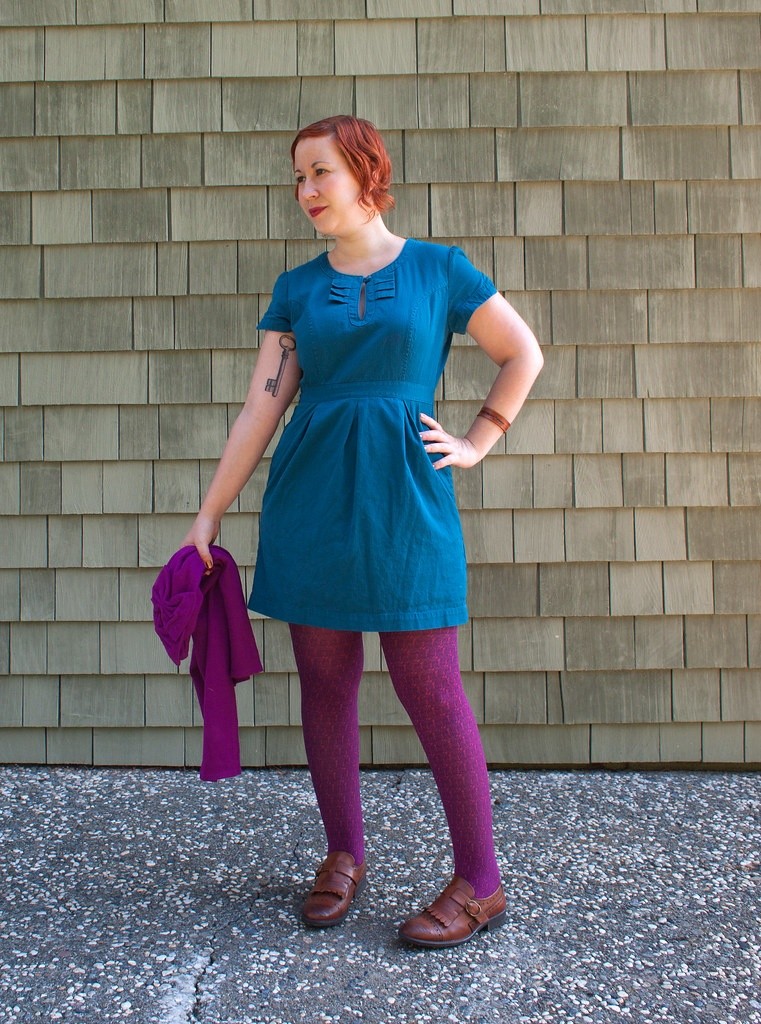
[474,406,512,438]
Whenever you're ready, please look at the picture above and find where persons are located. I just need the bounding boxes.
[180,116,547,950]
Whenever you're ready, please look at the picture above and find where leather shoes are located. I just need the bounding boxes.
[302,852,367,927]
[398,877,507,947]
